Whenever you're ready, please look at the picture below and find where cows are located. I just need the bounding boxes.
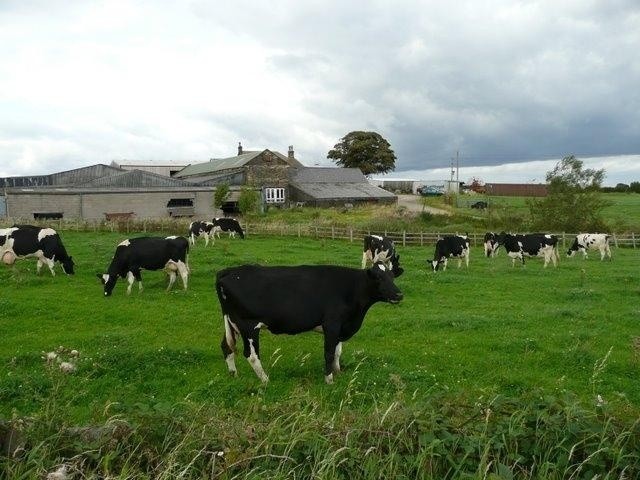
[97,236,191,296]
[212,218,244,240]
[427,231,470,274]
[215,261,404,385]
[566,234,613,262]
[361,234,400,270]
[0,227,75,277]
[186,220,215,248]
[483,231,560,269]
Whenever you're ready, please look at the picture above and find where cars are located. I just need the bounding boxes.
[417,186,444,195]
